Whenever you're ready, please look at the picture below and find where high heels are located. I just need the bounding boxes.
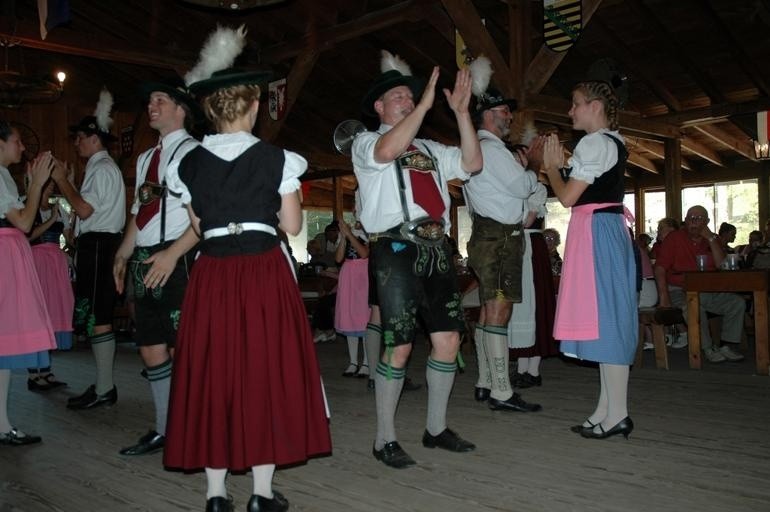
[580,416,634,441]
[572,418,592,432]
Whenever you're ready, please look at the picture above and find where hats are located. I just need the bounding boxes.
[188,67,272,94]
[361,70,416,118]
[67,113,118,141]
[469,85,517,119]
[136,72,204,117]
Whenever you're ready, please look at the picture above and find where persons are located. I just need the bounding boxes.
[717,223,736,254]
[309,272,337,344]
[363,233,422,392]
[462,55,545,414]
[114,22,248,460]
[0,118,55,447]
[628,226,658,351]
[544,229,562,276]
[23,171,75,393]
[336,210,371,378]
[543,81,639,440]
[733,230,763,261]
[763,219,770,246]
[638,234,652,254]
[163,51,333,512]
[50,91,127,409]
[351,48,483,470]
[297,221,341,270]
[659,217,688,345]
[506,144,557,388]
[654,206,745,363]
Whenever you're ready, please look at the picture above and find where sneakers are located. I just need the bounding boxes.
[719,345,744,362]
[643,342,654,352]
[313,328,336,343]
[27,377,58,393]
[671,331,688,352]
[41,372,67,388]
[343,363,359,376]
[666,334,672,346]
[353,364,369,378]
[704,345,725,363]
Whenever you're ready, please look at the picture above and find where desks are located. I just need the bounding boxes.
[684,268,770,375]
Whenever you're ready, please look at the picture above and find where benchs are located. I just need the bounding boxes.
[631,306,724,370]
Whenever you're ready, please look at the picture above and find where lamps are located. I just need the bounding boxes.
[56,71,66,92]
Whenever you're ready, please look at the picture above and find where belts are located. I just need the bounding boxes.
[643,277,655,281]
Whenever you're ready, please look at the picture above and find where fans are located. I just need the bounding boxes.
[332,119,368,159]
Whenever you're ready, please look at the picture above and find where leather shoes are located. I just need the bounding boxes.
[206,493,234,512]
[119,429,167,457]
[68,384,95,402]
[403,377,422,392]
[0,426,42,446]
[511,371,542,389]
[66,382,118,410]
[246,488,289,512]
[372,438,416,469]
[475,386,491,401]
[488,391,543,413]
[508,373,520,383]
[368,379,375,392]
[422,427,476,452]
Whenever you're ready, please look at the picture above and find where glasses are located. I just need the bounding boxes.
[491,107,509,113]
[688,215,704,221]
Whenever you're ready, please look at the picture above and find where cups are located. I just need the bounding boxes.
[719,260,730,272]
[696,255,707,272]
[726,253,739,270]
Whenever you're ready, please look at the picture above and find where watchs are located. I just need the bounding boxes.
[709,234,717,243]
